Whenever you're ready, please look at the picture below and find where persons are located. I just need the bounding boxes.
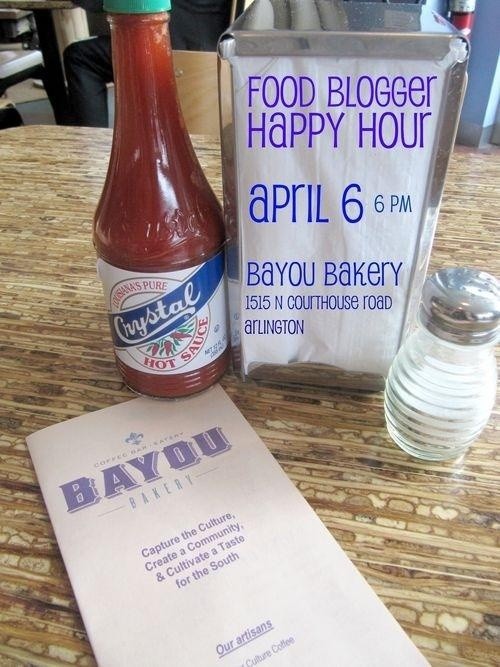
[63,0,247,126]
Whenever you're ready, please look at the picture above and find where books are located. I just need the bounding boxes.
[23,380,430,662]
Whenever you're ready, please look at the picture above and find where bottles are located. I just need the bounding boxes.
[93,1,231,400]
[381,269,500,465]
